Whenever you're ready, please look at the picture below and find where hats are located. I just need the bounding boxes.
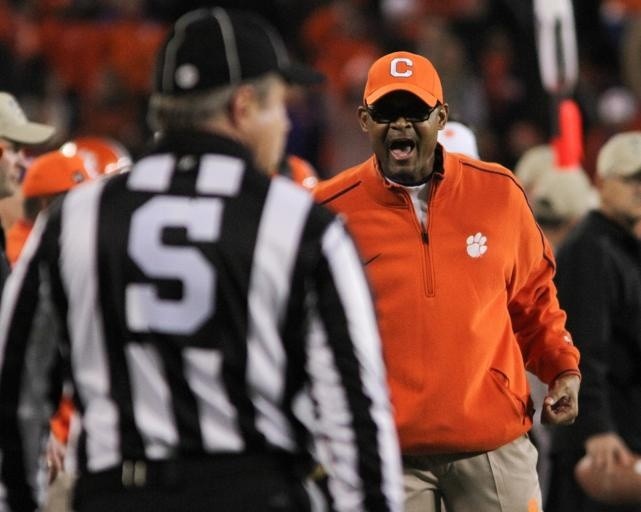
[156,4,325,93]
[363,51,443,107]
[597,131,640,177]
[22,136,130,201]
[0,92,55,144]
[515,143,592,220]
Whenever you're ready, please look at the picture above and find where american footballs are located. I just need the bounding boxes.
[573,455,641,507]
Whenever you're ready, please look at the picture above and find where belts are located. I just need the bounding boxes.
[75,452,252,492]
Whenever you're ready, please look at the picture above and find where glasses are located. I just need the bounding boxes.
[365,104,439,123]
